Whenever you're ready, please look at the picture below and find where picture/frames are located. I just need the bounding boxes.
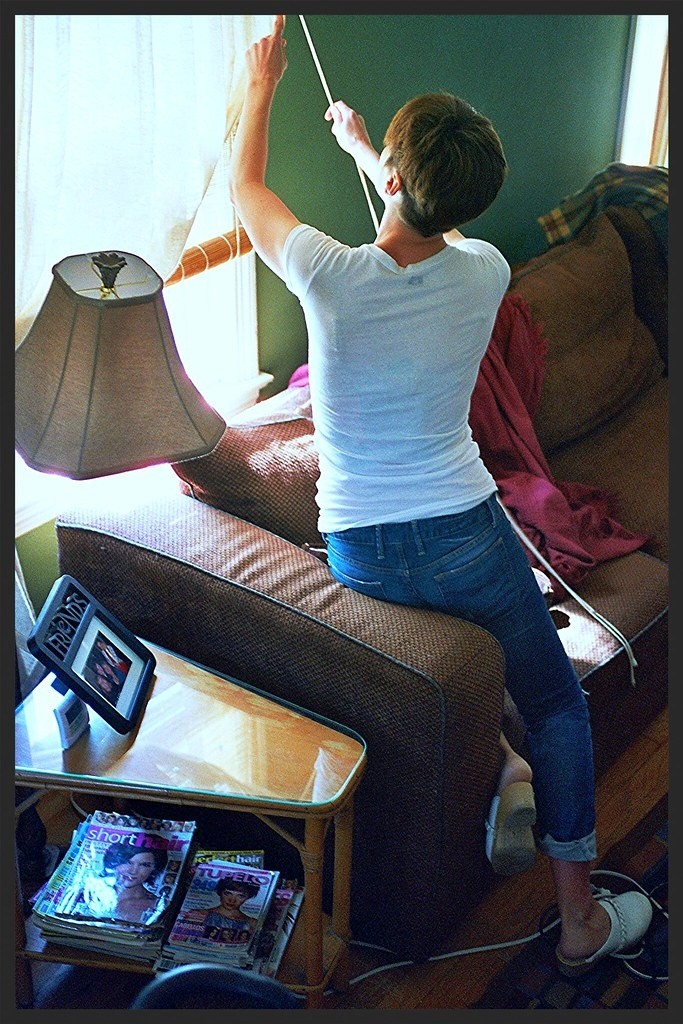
[29,575,157,736]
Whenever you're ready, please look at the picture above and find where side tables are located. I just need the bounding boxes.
[13,635,371,1007]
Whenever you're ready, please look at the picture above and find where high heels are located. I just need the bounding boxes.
[556,891,653,967]
[485,781,537,876]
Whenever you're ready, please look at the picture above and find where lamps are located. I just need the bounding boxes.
[13,249,229,480]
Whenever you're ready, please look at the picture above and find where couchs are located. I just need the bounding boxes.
[56,166,679,946]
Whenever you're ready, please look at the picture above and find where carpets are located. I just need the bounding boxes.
[467,797,669,1011]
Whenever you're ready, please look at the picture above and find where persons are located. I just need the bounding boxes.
[152,819,162,831]
[221,929,231,941]
[238,930,249,941]
[140,818,149,829]
[185,822,193,832]
[94,638,130,707]
[229,15,652,977]
[182,871,258,930]
[115,817,125,827]
[81,832,168,921]
[96,812,104,822]
[105,814,115,826]
[206,926,219,941]
[128,815,138,828]
[162,821,171,832]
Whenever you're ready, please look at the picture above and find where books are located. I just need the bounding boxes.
[29,809,305,984]
